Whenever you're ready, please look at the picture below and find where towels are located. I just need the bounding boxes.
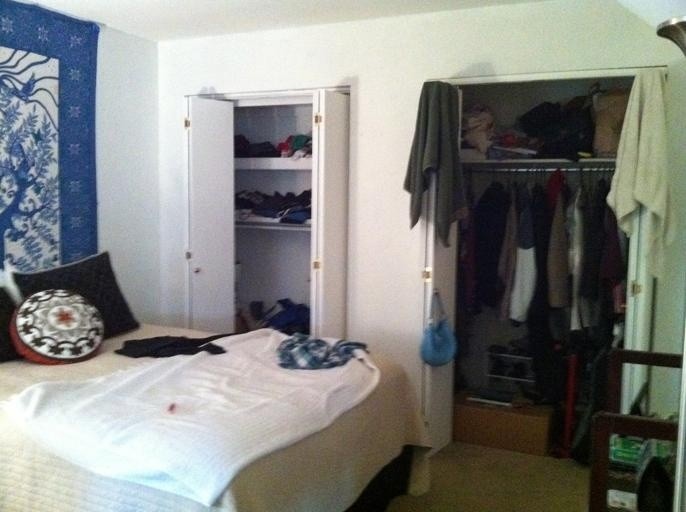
[606,70,677,281]
[405,80,468,247]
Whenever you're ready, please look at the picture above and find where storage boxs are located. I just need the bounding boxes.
[451,388,554,458]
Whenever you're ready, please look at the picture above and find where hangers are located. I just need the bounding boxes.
[481,162,623,197]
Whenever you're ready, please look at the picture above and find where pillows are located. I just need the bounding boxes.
[9,289,107,364]
[0,288,29,364]
[11,251,139,339]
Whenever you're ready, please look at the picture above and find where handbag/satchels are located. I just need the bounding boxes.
[420,318,456,366]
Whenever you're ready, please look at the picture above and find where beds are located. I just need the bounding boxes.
[0,326,412,512]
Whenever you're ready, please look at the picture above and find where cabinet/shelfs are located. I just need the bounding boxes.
[414,70,665,459]
[183,88,349,343]
